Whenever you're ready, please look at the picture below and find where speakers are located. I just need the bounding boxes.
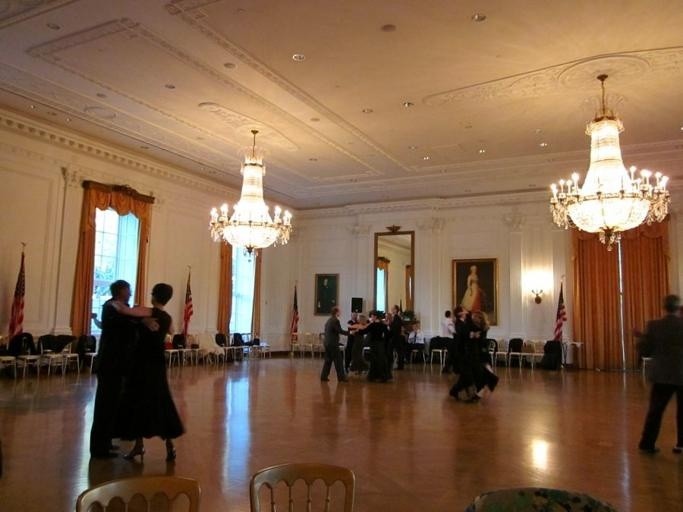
[351,297,363,313]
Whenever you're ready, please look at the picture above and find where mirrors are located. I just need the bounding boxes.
[373,226,415,322]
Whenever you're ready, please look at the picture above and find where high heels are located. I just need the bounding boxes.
[124,446,176,458]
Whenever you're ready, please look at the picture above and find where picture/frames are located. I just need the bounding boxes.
[315,274,339,316]
[452,258,498,325]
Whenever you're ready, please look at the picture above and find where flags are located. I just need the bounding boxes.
[184,271,193,337]
[8,251,25,345]
[290,282,300,343]
[554,282,567,344]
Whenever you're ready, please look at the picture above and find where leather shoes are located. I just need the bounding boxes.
[642,446,683,453]
[322,378,349,382]
[93,445,118,458]
[450,390,480,402]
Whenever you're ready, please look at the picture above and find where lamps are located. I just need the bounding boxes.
[550,74,672,252]
[209,130,292,257]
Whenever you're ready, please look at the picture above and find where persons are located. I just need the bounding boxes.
[318,304,404,384]
[440,304,499,407]
[636,294,682,456]
[215,327,227,350]
[90,280,187,461]
[460,264,481,315]
[317,278,336,312]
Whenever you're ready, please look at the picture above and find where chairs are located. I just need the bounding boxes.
[0,333,271,379]
[249,462,356,511]
[76,475,203,511]
[289,331,584,370]
[464,487,620,512]
